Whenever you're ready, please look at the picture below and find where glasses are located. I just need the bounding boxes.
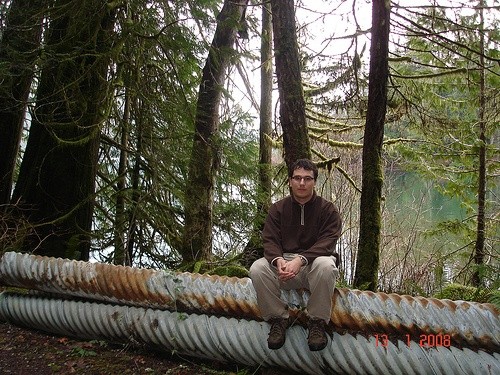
[291,175,314,182]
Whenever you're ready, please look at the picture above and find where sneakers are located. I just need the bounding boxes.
[267,318,289,350]
[307,318,328,351]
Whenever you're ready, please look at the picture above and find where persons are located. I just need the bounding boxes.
[249,158,342,351]
